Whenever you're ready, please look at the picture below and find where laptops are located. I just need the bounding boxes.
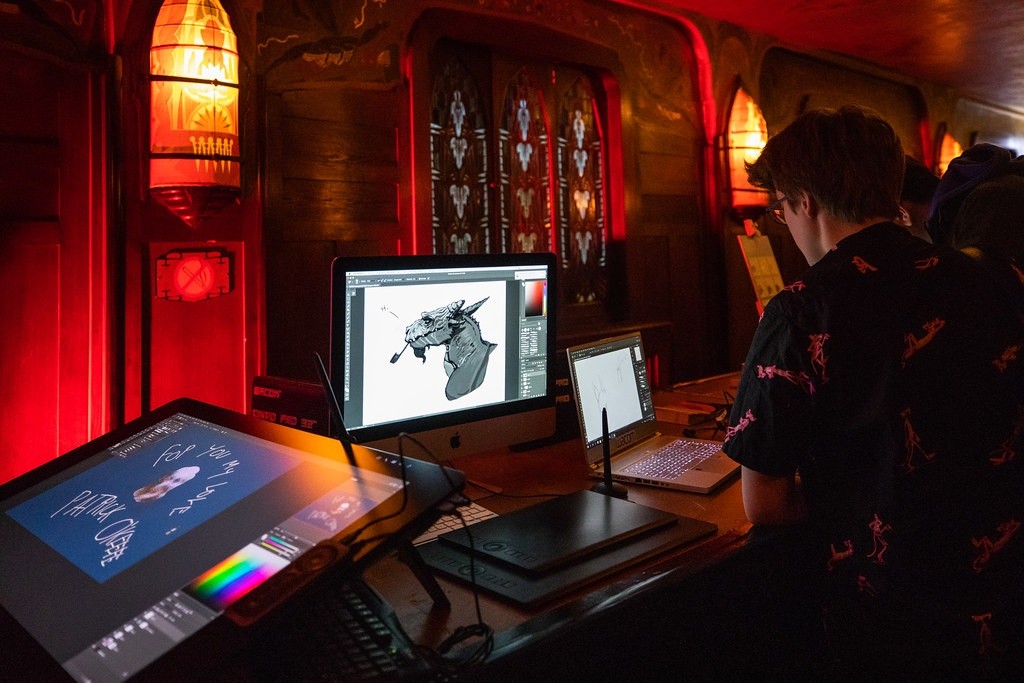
[567,332,742,494]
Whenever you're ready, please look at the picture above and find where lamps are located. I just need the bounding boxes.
[932,124,964,179]
[138,0,251,235]
[726,74,772,222]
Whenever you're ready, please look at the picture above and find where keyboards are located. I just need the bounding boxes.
[413,501,500,547]
[239,589,450,683]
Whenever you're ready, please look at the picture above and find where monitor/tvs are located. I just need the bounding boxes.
[0,395,467,683]
[328,252,558,463]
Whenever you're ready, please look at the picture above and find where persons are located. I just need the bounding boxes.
[721,103,1024,683]
[893,142,1024,282]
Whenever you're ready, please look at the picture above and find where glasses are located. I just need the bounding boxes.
[766,185,800,224]
